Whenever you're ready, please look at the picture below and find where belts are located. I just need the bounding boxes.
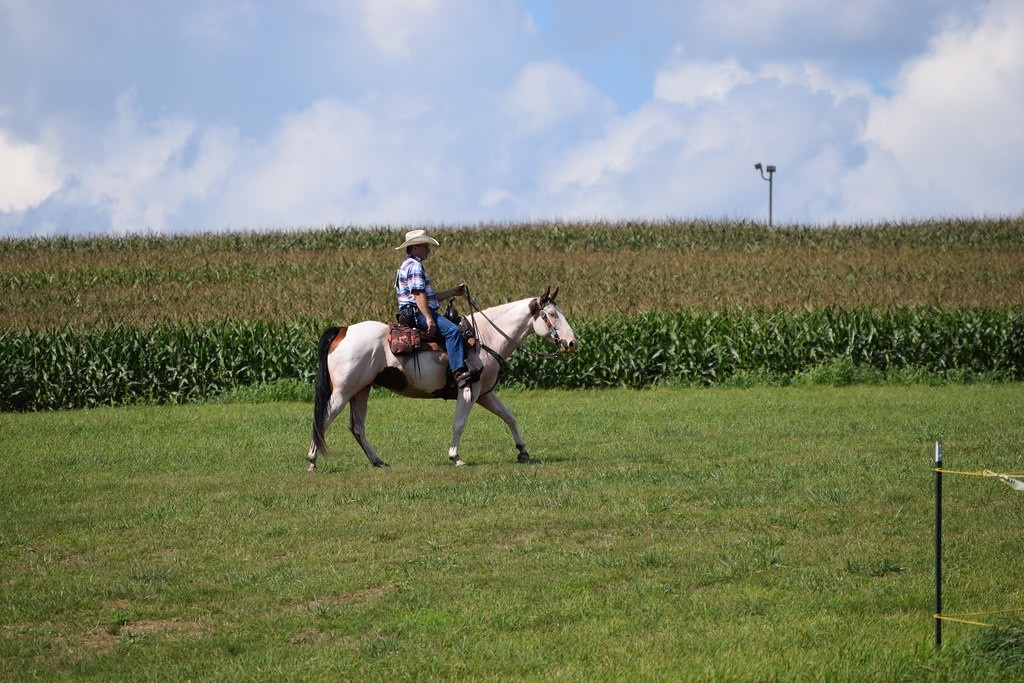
[400,304,413,310]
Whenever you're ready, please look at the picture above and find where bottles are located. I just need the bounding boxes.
[411,313,421,349]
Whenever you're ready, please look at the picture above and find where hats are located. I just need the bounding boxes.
[395,229,439,250]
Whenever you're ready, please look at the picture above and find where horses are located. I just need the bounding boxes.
[304,285,578,473]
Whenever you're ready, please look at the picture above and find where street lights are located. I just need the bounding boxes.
[755,162,776,228]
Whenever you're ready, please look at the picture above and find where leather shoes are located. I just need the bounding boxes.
[454,366,484,388]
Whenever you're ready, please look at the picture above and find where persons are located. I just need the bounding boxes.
[394,230,484,389]
